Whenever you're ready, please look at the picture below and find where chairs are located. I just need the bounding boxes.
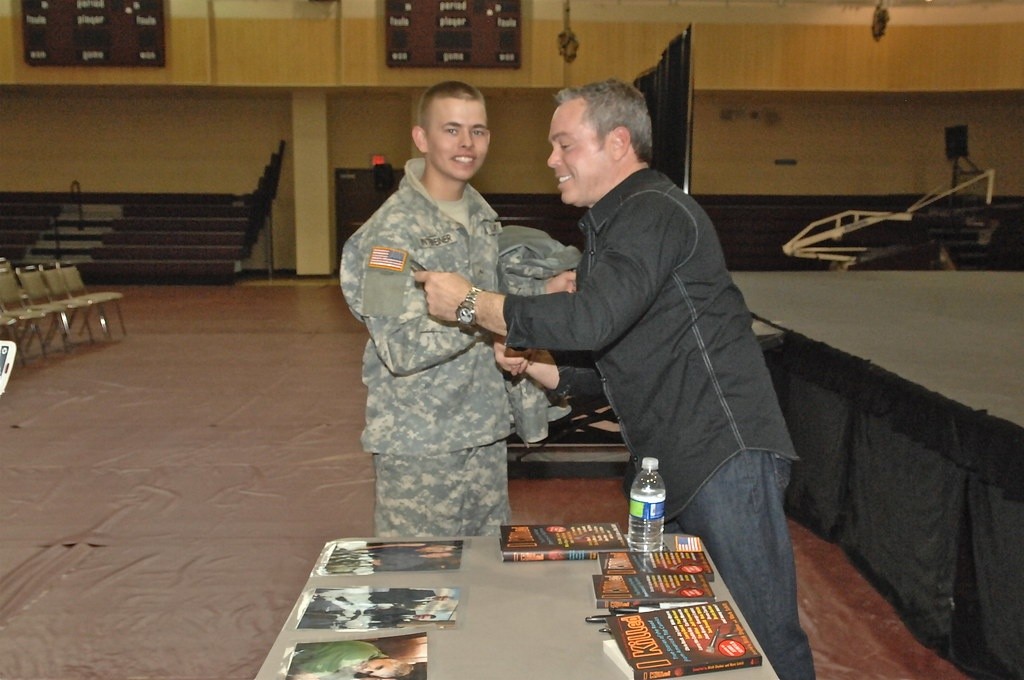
[16,265,76,356]
[39,262,110,349]
[0,314,26,367]
[0,269,55,364]
[56,260,128,342]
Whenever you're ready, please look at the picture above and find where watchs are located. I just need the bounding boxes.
[455,286,485,328]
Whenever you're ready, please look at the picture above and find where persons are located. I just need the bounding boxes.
[415,78,817,680]
[340,82,580,539]
[286,540,458,680]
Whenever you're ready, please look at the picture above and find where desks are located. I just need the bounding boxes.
[253,530,780,680]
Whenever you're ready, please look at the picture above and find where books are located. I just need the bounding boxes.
[597,551,715,582]
[602,600,762,680]
[591,573,717,609]
[498,520,630,563]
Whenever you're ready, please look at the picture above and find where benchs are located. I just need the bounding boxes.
[479,193,583,247]
[693,192,1024,272]
[0,192,252,285]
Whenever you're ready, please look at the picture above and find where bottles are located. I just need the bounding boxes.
[626,457,666,551]
